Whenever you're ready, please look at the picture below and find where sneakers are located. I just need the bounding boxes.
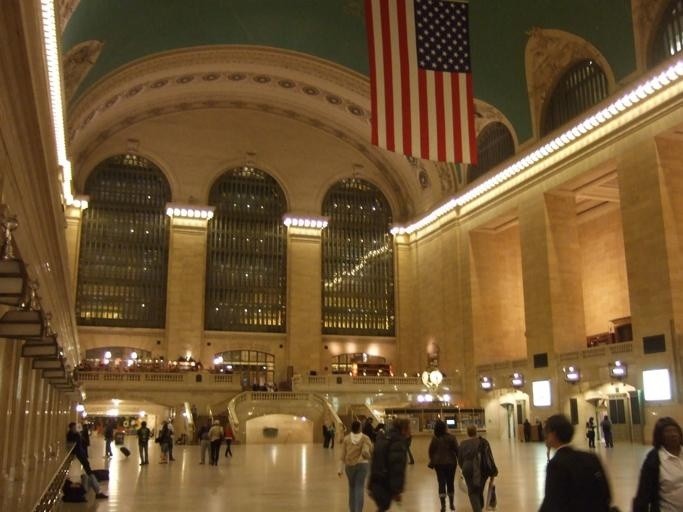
[95,490,109,500]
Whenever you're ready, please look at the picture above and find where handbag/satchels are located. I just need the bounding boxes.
[361,435,371,459]
[63,479,87,502]
[478,436,497,477]
[485,480,497,511]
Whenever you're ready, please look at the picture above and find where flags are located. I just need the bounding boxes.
[364,0,479,168]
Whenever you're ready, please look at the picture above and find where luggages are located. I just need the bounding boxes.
[120,446,131,457]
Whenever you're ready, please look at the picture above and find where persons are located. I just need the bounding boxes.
[456,422,493,512]
[61,472,109,499]
[328,421,336,448]
[426,419,460,512]
[66,418,235,475]
[522,418,531,441]
[361,416,415,511]
[336,420,374,511]
[530,413,613,512]
[584,416,596,448]
[599,414,613,448]
[321,420,330,448]
[629,414,682,512]
[535,417,542,441]
